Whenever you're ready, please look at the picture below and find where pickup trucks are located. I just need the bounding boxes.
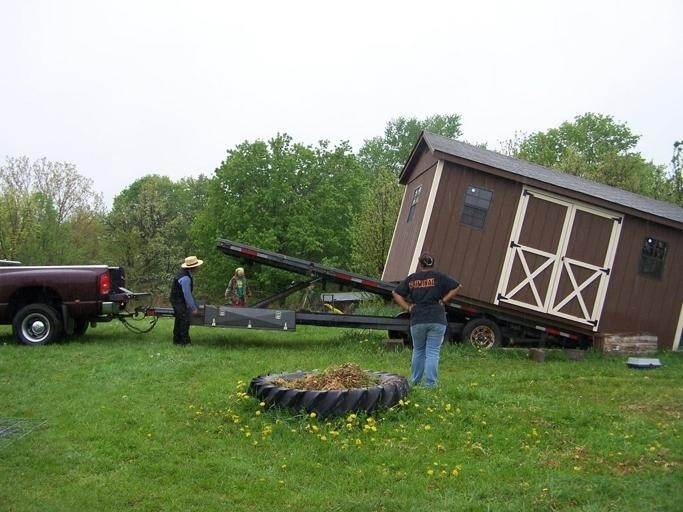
[0,259,149,346]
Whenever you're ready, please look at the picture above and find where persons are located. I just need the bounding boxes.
[387,254,461,386]
[168,255,204,345]
[223,266,250,309]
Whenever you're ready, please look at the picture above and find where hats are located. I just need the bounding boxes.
[180,255,204,268]
